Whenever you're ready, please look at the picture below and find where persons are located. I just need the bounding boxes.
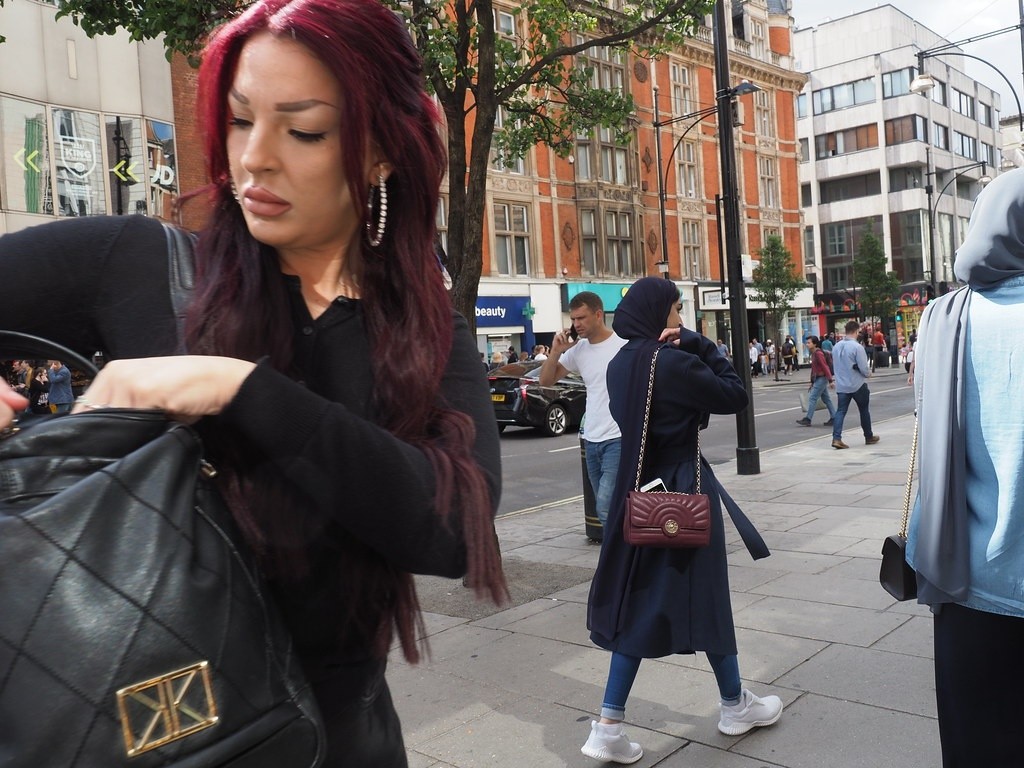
[831,321,880,449]
[717,338,730,361]
[901,336,917,417]
[906,168,1024,768]
[749,335,800,378]
[818,327,884,375]
[581,276,783,762]
[0,0,512,768]
[796,335,836,426]
[539,293,629,543]
[477,344,564,373]
[9,360,74,414]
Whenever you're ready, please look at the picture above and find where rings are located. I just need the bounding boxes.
[75,395,102,409]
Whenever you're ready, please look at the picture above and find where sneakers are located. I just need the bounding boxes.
[718,688,784,735]
[581,720,643,764]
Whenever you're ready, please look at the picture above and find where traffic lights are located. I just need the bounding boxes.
[896,310,902,322]
[939,281,948,298]
[926,284,935,305]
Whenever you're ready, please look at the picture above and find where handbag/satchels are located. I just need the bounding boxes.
[623,490,711,546]
[0,333,330,768]
[799,386,828,411]
[878,533,917,602]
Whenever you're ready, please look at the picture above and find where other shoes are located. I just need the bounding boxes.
[823,418,835,426]
[866,436,880,444]
[796,417,812,426]
[832,438,850,448]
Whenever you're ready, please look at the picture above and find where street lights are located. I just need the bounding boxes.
[710,0,763,475]
[908,0,1024,131]
[923,145,993,307]
[652,84,720,283]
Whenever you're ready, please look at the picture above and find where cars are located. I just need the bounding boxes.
[486,360,588,438]
